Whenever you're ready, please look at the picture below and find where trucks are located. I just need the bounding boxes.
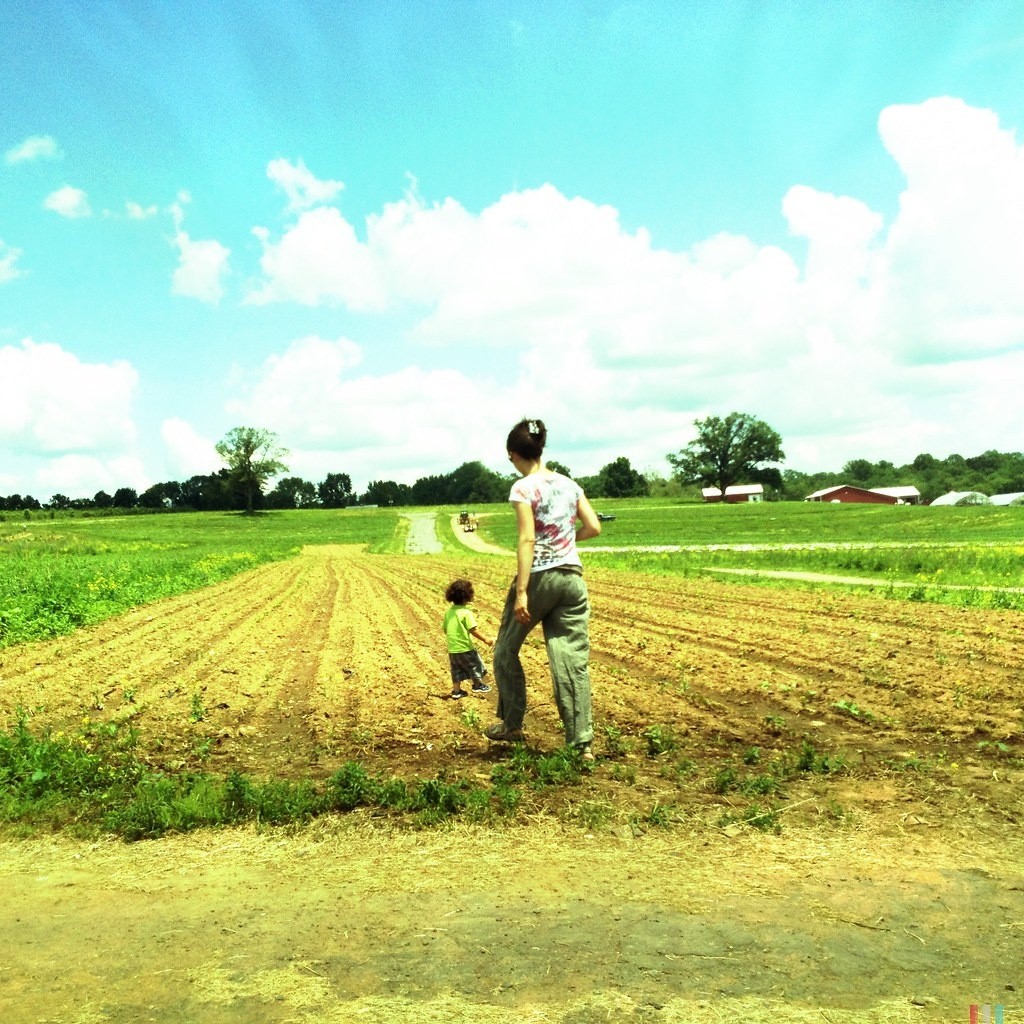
[597,513,615,521]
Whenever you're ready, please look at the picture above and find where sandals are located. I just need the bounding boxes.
[578,750,594,765]
[483,723,526,743]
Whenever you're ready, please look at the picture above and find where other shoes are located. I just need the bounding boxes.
[452,689,467,698]
[471,683,491,692]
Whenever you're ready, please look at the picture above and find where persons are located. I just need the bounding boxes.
[484,419,601,762]
[440,580,493,699]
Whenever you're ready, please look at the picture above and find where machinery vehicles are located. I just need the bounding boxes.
[458,510,475,532]
